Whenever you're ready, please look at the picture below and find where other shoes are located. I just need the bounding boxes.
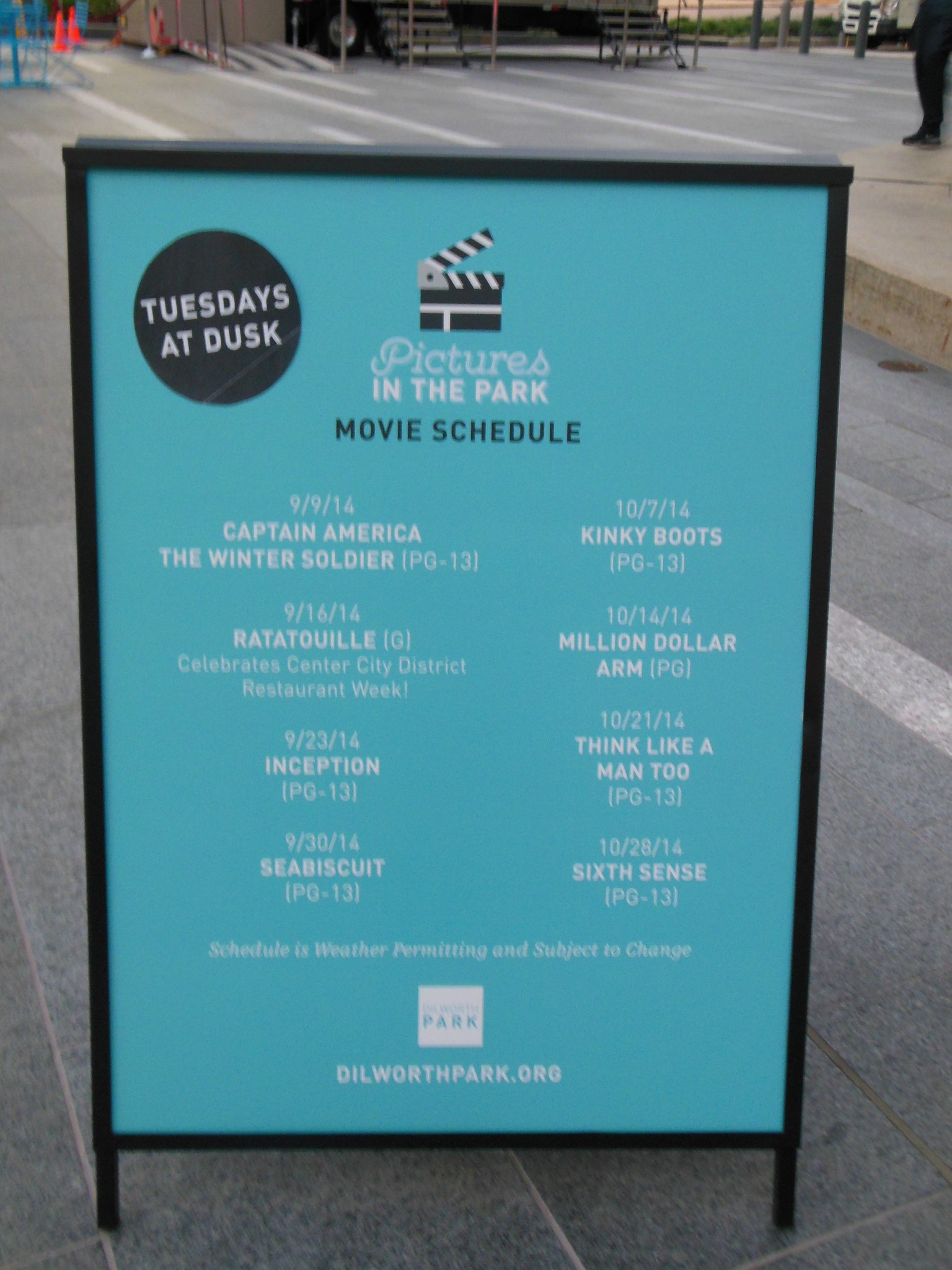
[903,130,942,149]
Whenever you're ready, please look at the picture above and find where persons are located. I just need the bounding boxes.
[902,0,952,147]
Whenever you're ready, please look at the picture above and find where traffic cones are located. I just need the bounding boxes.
[52,9,72,54]
[68,6,85,46]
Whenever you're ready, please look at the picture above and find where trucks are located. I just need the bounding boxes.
[842,0,922,51]
